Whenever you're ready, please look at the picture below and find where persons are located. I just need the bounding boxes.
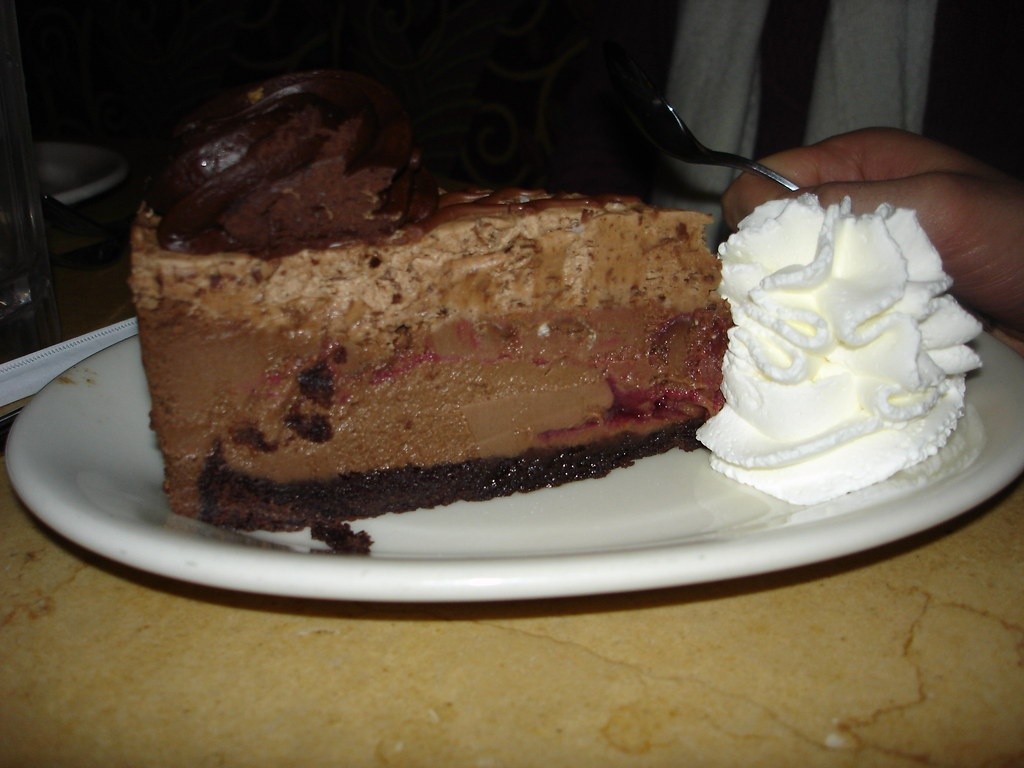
[557,0,1024,319]
[718,128,1024,361]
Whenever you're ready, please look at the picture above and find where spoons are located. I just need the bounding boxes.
[600,36,798,193]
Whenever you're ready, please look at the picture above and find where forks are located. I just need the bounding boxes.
[40,192,135,238]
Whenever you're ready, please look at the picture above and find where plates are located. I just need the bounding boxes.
[6,327,1024,603]
[33,139,131,205]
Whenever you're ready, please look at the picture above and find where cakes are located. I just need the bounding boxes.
[125,66,984,533]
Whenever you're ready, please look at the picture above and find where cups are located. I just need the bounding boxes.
[0,0,55,353]
